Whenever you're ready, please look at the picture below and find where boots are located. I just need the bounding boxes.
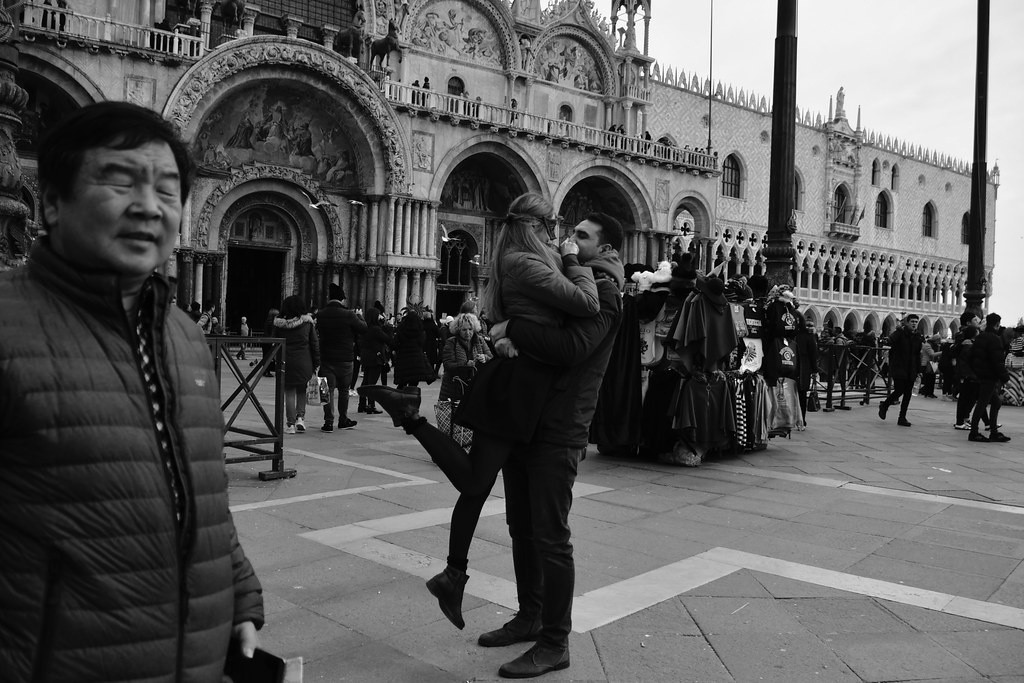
[426,566,469,630]
[357,385,422,428]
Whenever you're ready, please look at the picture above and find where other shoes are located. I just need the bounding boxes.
[879,402,888,420]
[968,433,989,442]
[358,405,382,414]
[955,420,972,430]
[925,394,937,398]
[989,432,1011,442]
[349,389,358,396]
[898,417,911,426]
[985,422,1002,430]
[943,393,959,401]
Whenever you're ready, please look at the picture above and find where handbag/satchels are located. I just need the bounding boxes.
[926,360,940,375]
[807,392,821,412]
[444,367,474,399]
[433,401,474,450]
[305,377,331,407]
[364,353,390,374]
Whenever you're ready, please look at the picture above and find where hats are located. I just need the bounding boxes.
[328,282,347,301]
[696,274,728,305]
[440,316,454,324]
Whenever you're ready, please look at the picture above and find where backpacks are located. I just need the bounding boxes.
[837,336,856,370]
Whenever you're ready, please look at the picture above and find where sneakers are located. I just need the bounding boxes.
[338,418,358,430]
[477,612,543,647]
[498,633,571,680]
[284,417,305,434]
[320,424,333,433]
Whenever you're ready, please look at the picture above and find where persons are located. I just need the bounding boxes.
[953,311,1024,442]
[271,294,320,434]
[236,316,249,360]
[666,140,705,166]
[158,18,172,32]
[644,130,651,154]
[438,301,493,401]
[878,314,923,426]
[263,300,454,396]
[357,306,395,415]
[607,124,626,149]
[806,321,889,389]
[478,212,627,679]
[179,301,223,335]
[314,284,368,433]
[412,76,430,106]
[392,309,437,388]
[921,334,942,398]
[0,100,265,683]
[355,193,600,630]
